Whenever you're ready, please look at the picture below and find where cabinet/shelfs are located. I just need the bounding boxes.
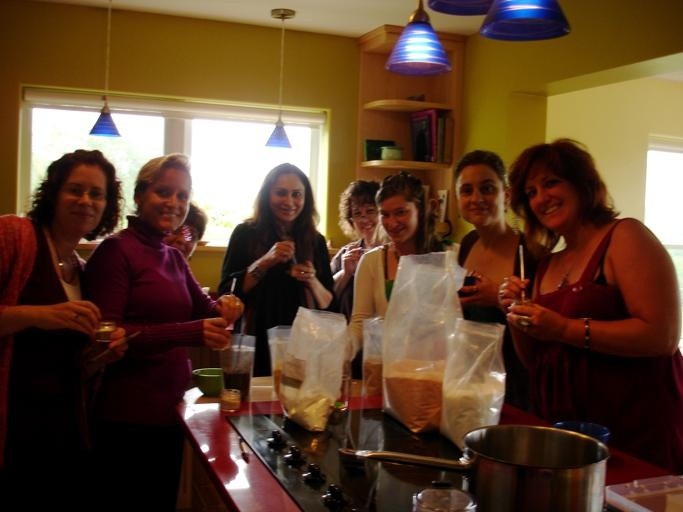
[352,23,468,244]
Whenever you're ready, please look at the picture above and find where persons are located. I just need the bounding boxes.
[176,203,207,260]
[442,149,538,411]
[83,156,247,512]
[331,179,391,377]
[345,174,460,379]
[498,140,682,478]
[217,162,336,376]
[0,150,130,512]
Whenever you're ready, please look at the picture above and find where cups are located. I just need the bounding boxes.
[223,333,254,405]
[456,275,479,298]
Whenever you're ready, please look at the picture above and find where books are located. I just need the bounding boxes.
[410,110,454,167]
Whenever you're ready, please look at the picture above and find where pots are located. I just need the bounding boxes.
[337,423,610,511]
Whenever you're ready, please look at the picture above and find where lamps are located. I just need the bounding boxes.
[476,0,572,44]
[87,1,123,138]
[383,0,454,78]
[426,0,496,16]
[260,7,298,150]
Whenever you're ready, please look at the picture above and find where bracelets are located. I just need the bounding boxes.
[584,317,592,352]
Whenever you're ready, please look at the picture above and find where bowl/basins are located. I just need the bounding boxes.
[380,147,403,160]
[191,366,225,396]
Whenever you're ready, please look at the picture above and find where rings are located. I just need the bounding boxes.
[528,320,533,328]
[235,303,241,308]
[74,313,81,320]
[473,285,478,293]
[300,271,305,276]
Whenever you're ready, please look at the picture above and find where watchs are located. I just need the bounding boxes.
[247,260,263,280]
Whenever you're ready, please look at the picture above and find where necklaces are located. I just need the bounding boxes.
[53,238,77,284]
[554,224,599,288]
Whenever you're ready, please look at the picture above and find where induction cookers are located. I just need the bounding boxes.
[225,406,477,511]
